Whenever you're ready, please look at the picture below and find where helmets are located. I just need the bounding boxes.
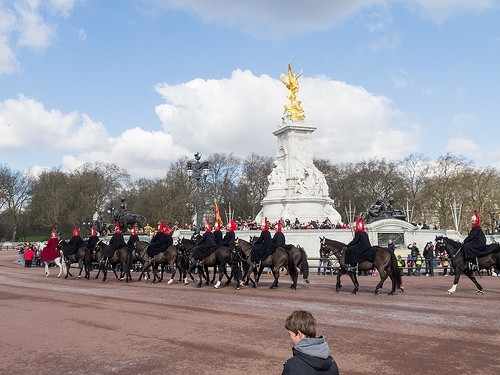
[50,227,59,239]
[114,221,121,234]
[164,223,170,235]
[73,225,79,236]
[353,217,361,233]
[91,227,97,236]
[275,220,281,233]
[204,218,212,232]
[214,219,221,231]
[261,217,269,231]
[471,209,481,226]
[157,219,164,233]
[131,227,138,235]
[359,218,365,232]
[226,220,237,233]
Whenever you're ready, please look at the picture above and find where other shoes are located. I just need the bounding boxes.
[196,259,204,265]
[254,262,259,266]
[468,259,475,270]
[62,259,70,262]
[99,259,106,262]
[348,268,357,272]
[147,258,154,263]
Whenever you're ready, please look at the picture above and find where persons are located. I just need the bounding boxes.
[250,217,275,261]
[222,219,237,248]
[17,215,500,274]
[147,220,166,256]
[87,227,99,253]
[164,224,174,248]
[39,227,59,261]
[127,228,139,252]
[25,247,34,267]
[273,220,286,246]
[193,219,217,260]
[103,221,127,258]
[213,218,222,243]
[281,311,340,375]
[63,225,84,255]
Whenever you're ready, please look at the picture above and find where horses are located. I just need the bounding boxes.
[317,234,405,298]
[35,236,311,292]
[431,234,500,297]
